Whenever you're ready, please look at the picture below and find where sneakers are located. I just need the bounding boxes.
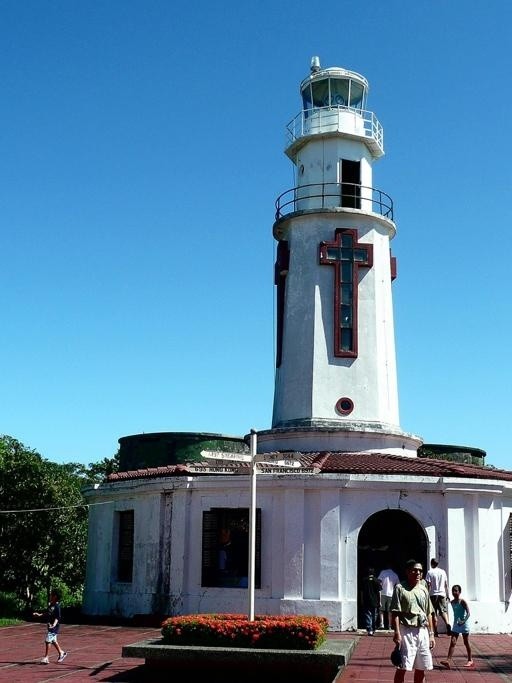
[440,661,450,670]
[446,624,451,636]
[57,652,67,663]
[464,660,473,667]
[434,632,438,637]
[368,624,392,636]
[40,658,49,664]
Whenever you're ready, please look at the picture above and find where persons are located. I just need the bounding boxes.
[33,587,68,663]
[439,584,474,668]
[388,559,436,682]
[358,554,452,638]
